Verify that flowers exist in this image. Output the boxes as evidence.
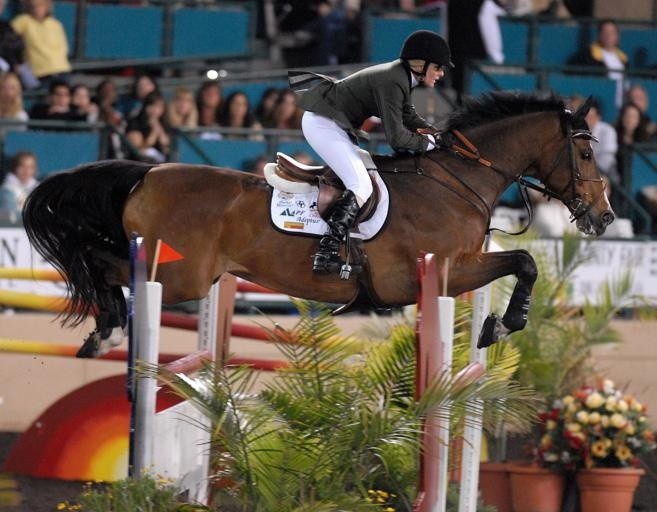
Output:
[529,377,656,474]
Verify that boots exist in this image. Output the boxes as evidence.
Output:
[312,189,362,275]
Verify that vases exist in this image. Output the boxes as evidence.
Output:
[575,464,645,512]
[505,462,566,511]
[479,458,512,512]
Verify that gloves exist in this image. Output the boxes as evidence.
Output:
[433,131,456,149]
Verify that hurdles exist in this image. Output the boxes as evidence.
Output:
[417,284,493,512]
[127,280,220,508]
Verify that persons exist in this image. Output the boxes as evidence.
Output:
[0,0,656,242]
[301,30,456,274]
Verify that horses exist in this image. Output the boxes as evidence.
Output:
[20,87,616,358]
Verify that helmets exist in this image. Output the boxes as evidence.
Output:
[401,30,455,68]
